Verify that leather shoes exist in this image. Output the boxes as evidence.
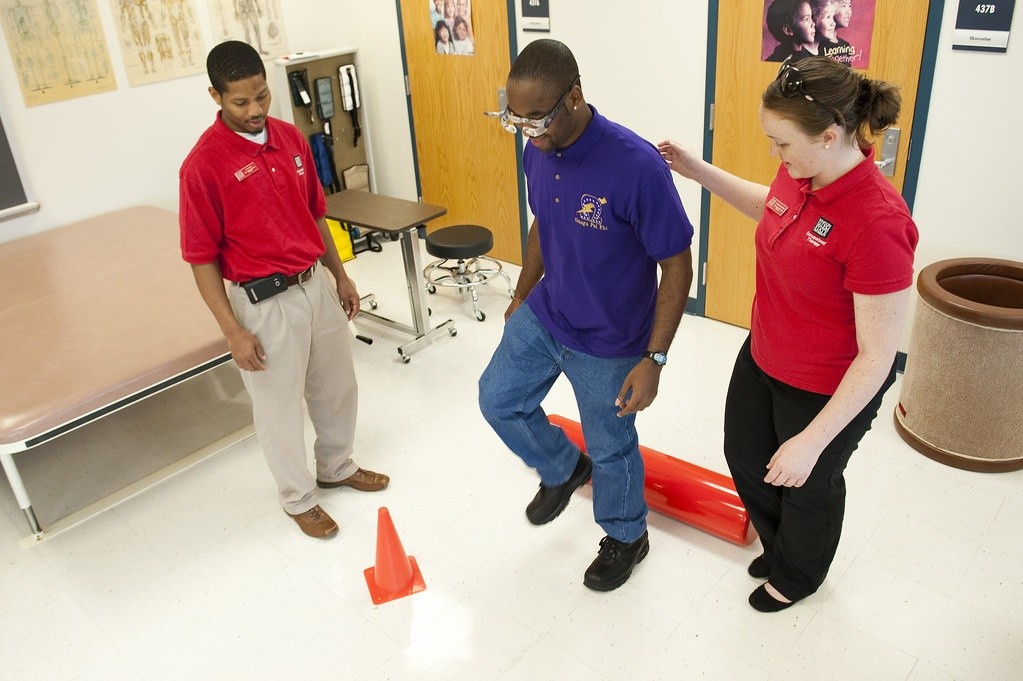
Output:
[284,505,339,539]
[526,453,592,524]
[316,467,390,491]
[583,529,649,591]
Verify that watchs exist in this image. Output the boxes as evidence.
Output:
[643,351,666,366]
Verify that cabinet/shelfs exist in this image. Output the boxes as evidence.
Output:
[273,46,385,255]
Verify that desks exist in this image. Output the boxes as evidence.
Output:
[1,206,261,547]
[324,189,457,363]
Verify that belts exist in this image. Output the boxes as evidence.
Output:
[234,261,318,289]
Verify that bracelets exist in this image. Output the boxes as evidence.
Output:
[509,289,525,302]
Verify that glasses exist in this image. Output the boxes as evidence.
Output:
[776,54,840,125]
[501,75,581,136]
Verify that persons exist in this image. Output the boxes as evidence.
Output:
[179,39,390,540]
[656,54,919,613]
[452,18,475,55]
[817,0,853,66]
[433,1,467,25]
[803,1,837,58]
[432,21,456,55]
[477,41,693,594]
[765,0,815,64]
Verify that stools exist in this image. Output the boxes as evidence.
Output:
[422,224,517,321]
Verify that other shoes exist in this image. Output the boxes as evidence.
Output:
[749,581,796,613]
[748,553,771,578]
